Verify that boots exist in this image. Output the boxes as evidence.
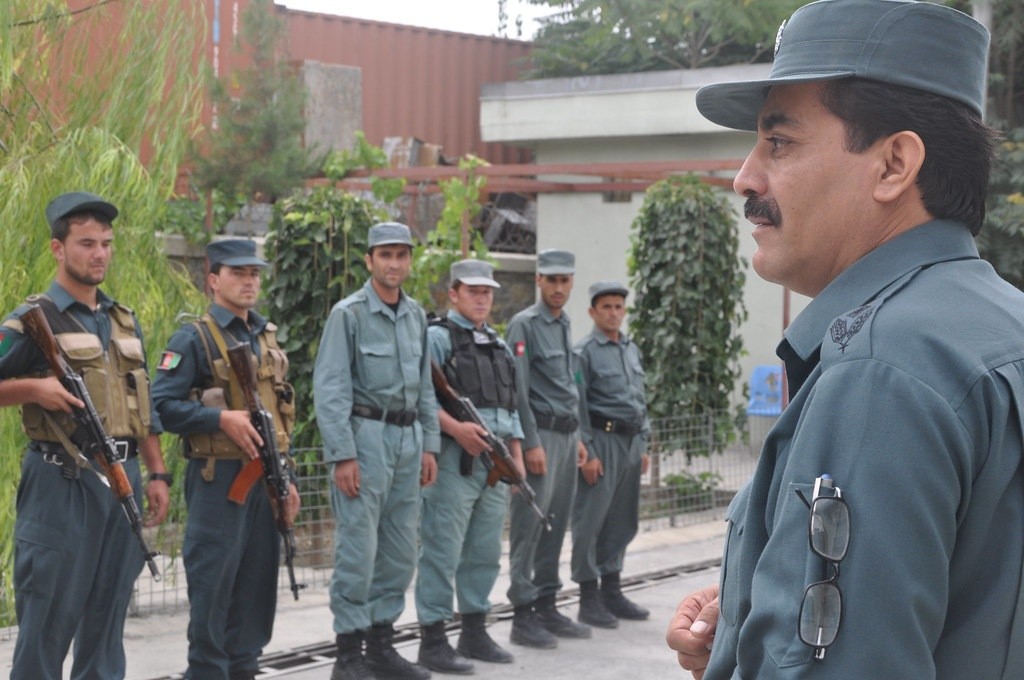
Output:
[510,604,559,647]
[363,623,431,680]
[578,581,620,629]
[329,628,380,680]
[600,574,649,620]
[456,613,515,664]
[418,620,473,674]
[535,594,592,639]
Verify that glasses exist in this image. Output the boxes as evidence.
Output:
[795,486,851,659]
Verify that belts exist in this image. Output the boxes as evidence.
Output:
[36,439,136,462]
[351,404,419,426]
[591,413,643,436]
[534,414,581,434]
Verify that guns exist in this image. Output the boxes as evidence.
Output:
[224,340,301,603]
[428,360,557,534]
[19,303,162,583]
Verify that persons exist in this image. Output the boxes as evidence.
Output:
[0,193,170,680]
[416,259,524,677]
[503,249,589,649]
[315,222,442,680]
[667,0,1024,679]
[574,281,651,628]
[151,239,301,680]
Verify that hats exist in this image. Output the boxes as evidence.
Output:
[695,0,991,130]
[367,223,416,251]
[451,260,500,288]
[45,192,119,230]
[589,282,628,306]
[206,239,269,266]
[537,250,576,275]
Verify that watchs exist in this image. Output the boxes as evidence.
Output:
[148,473,174,486]
